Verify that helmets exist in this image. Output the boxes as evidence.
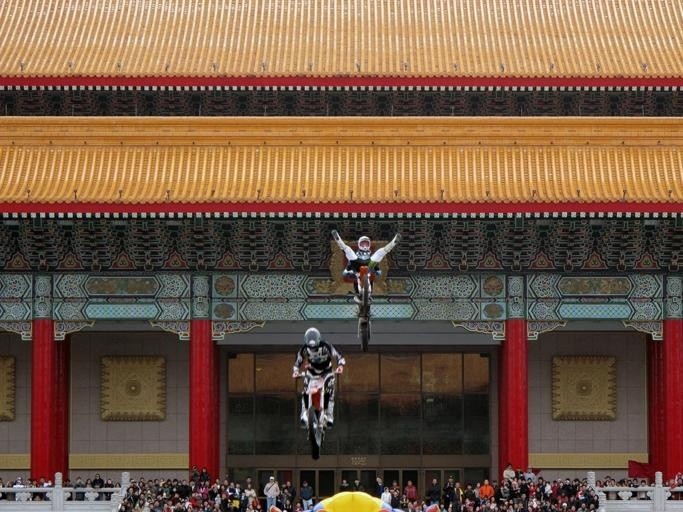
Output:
[358,236,370,251]
[304,328,320,347]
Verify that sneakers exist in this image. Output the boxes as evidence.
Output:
[332,230,341,241]
[394,234,401,244]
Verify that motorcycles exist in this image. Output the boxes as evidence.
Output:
[289,367,341,460]
[342,266,382,352]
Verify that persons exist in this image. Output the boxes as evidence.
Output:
[341,463,599,512]
[596,472,682,500]
[0,466,314,512]
[329,227,402,303]
[290,327,346,430]
